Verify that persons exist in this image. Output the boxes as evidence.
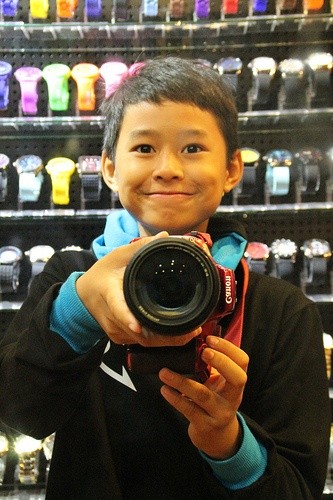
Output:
[0,54,333,500]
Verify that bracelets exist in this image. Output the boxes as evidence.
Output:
[303,0,325,11]
[0,0,21,19]
[278,0,296,12]
[111,0,130,20]
[85,0,104,19]
[55,0,78,17]
[252,0,270,14]
[192,0,210,20]
[140,0,159,17]
[29,0,50,19]
[221,0,240,16]
[168,0,186,18]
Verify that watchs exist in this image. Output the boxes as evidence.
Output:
[129,63,145,76]
[42,431,57,486]
[69,63,99,112]
[13,66,42,113]
[97,60,128,103]
[306,50,333,94]
[13,431,42,487]
[212,56,243,96]
[40,62,72,112]
[0,433,9,488]
[246,56,274,106]
[0,149,332,311]
[321,331,333,382]
[0,62,15,110]
[278,56,306,105]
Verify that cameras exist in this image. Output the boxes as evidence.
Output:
[116,234,238,384]
